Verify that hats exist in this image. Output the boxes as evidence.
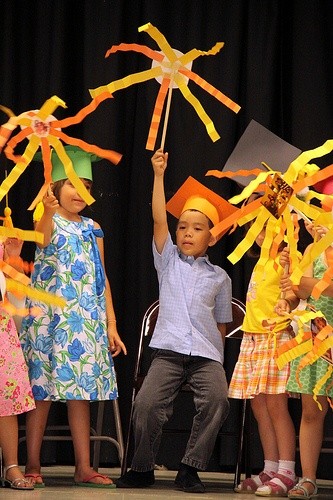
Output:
[31,145,103,183]
[166,176,241,246]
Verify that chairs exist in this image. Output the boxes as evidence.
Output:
[0,400,124,478]
[122,298,248,488]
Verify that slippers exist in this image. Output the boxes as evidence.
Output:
[75,473,117,488]
[24,473,45,488]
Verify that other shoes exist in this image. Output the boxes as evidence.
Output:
[173,467,206,492]
[115,470,155,488]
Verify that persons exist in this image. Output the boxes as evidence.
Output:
[226,191,301,497]
[115,148,239,493]
[0,227,37,489]
[24,145,128,488]
[274,174,333,498]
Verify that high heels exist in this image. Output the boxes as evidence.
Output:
[1,465,35,490]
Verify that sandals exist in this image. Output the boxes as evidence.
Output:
[233,471,276,493]
[254,473,299,497]
[288,478,319,499]
